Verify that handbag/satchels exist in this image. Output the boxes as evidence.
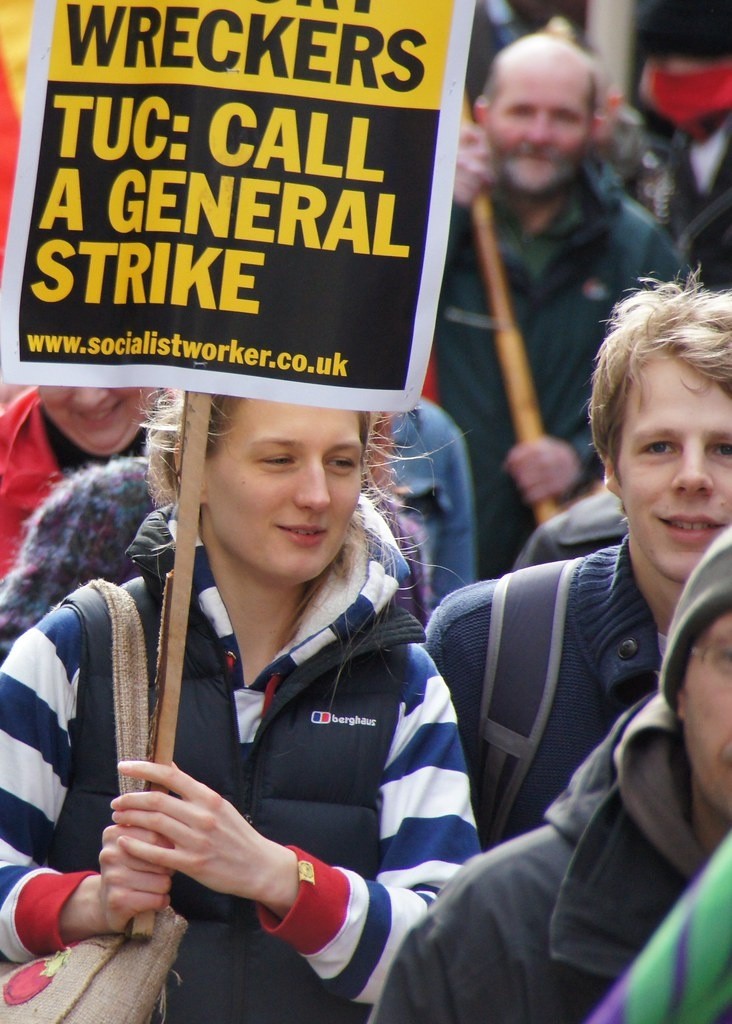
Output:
[0,576,187,1024]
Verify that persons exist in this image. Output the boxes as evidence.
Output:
[1,385,477,661]
[424,272,731,846]
[373,525,731,1024]
[432,31,702,573]
[0,390,473,1023]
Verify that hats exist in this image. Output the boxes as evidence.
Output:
[658,527,732,712]
[634,0,732,64]
